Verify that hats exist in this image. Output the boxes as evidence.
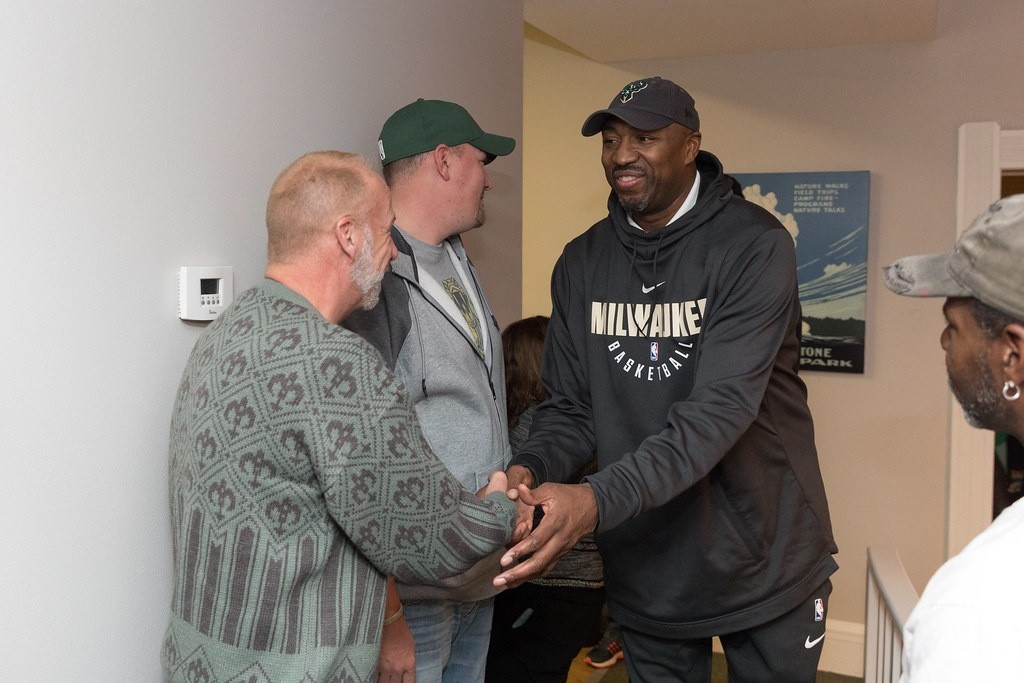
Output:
[377,98,516,166]
[882,194,1024,321]
[581,77,700,138]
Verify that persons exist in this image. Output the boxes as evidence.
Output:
[341,98,514,683]
[159,151,535,683]
[476,77,839,683]
[884,193,1024,683]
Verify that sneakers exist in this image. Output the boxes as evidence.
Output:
[584,622,624,667]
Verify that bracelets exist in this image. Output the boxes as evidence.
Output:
[383,602,403,627]
